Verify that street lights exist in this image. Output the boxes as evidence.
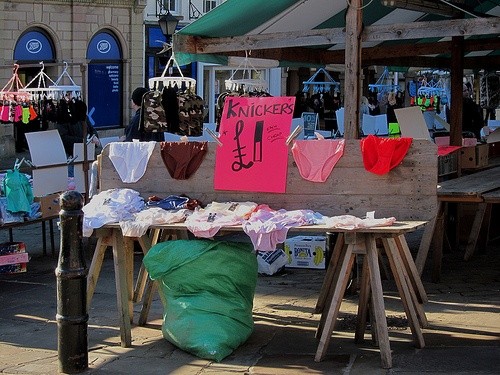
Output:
[159,10,180,76]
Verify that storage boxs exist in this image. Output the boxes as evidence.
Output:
[284,236,328,269]
[24,131,96,197]
[462,145,489,169]
[438,147,459,177]
[0,242,28,275]
[35,190,68,219]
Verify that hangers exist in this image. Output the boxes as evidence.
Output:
[417,78,446,97]
[224,50,270,93]
[148,52,197,89]
[303,68,341,93]
[368,66,402,94]
[0,61,82,105]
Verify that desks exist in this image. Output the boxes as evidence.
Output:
[415,167,500,278]
[57,222,428,369]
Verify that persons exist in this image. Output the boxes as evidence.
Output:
[123,87,166,142]
[462,84,483,132]
[57,115,101,159]
